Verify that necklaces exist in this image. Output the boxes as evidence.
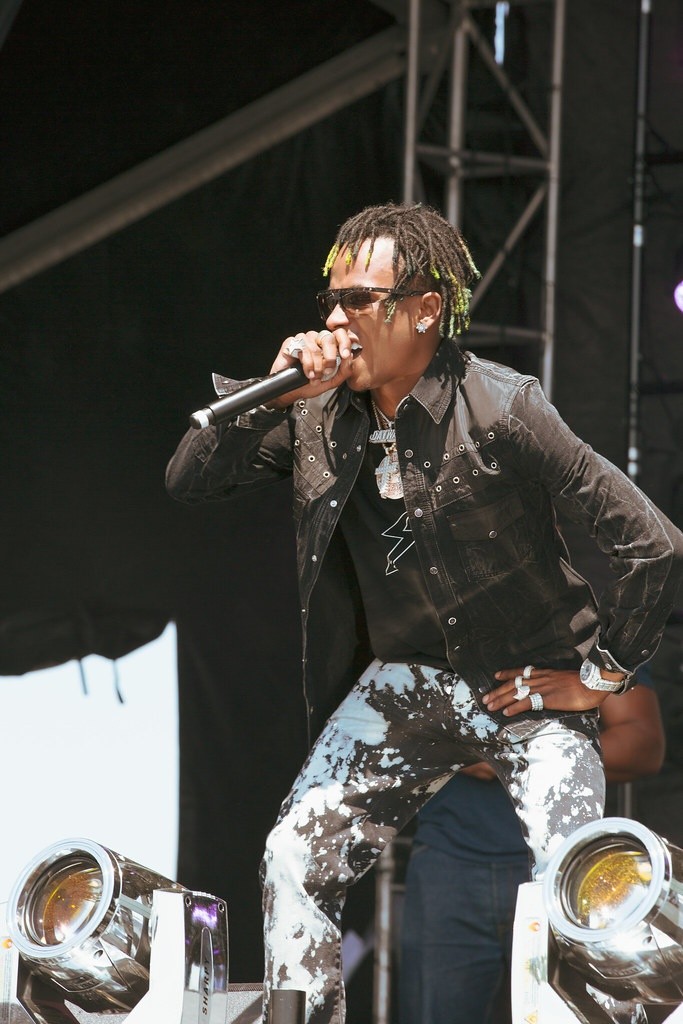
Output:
[368,399,405,499]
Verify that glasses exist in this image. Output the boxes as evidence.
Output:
[316,287,428,321]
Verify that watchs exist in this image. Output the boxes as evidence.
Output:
[580,658,624,692]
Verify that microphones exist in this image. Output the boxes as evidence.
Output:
[189,347,341,429]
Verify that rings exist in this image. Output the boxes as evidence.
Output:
[318,331,333,340]
[515,676,523,688]
[529,693,543,711]
[523,666,535,679]
[514,686,531,701]
[285,337,306,357]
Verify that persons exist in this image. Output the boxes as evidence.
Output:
[165,203,683,1024]
[396,509,665,1024]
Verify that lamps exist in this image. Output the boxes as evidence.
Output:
[510,817,683,1024]
[6,838,228,1024]
